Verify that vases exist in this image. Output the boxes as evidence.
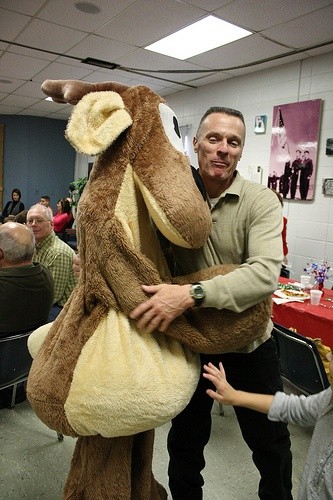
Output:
[316,281,324,290]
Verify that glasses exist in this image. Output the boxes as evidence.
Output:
[25,218,52,227]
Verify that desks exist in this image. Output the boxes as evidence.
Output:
[272,276,333,350]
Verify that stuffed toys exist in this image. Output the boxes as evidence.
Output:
[26,78,276,500]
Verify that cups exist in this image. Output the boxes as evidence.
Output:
[310,290,323,306]
[300,275,311,287]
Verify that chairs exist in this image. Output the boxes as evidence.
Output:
[272,322,330,396]
[0,329,64,442]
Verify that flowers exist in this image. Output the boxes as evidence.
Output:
[304,258,333,282]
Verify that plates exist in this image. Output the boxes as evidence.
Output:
[274,290,310,299]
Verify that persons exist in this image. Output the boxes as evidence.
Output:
[0,188,25,225]
[38,196,54,221]
[64,198,75,229]
[25,204,81,325]
[73,107,294,500]
[258,108,314,199]
[50,197,73,233]
[274,192,290,280]
[202,349,333,500]
[0,222,55,410]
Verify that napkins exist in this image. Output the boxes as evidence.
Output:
[287,281,314,289]
[273,297,304,305]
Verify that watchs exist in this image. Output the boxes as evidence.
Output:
[189,282,206,308]
